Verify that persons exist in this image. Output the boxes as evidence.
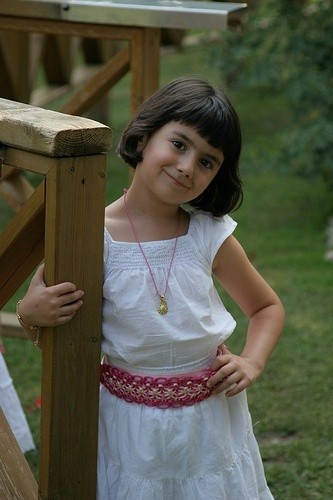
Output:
[16,79,285,500]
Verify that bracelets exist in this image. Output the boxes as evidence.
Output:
[16,300,40,347]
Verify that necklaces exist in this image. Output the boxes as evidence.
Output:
[123,189,180,315]
